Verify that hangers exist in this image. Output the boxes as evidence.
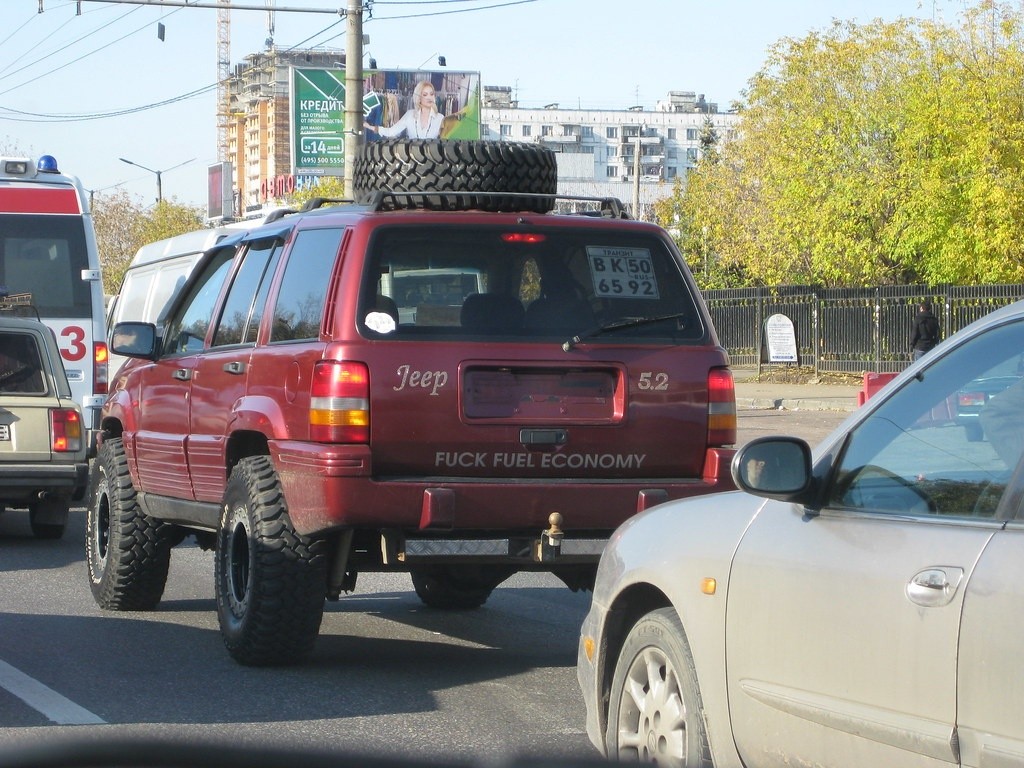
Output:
[370,88,457,102]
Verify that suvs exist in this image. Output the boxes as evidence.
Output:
[89,140,739,667]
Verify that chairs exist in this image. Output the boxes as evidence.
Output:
[461,293,523,329]
[367,293,398,333]
[525,281,597,335]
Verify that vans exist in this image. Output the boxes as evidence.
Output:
[1,154,249,500]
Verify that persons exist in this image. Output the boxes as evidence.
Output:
[908,300,941,361]
[745,457,766,488]
[363,81,445,140]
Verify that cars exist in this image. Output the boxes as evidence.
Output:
[0,319,89,540]
[578,298,1024,768]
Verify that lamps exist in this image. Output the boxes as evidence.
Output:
[418,51,446,71]
[362,52,377,69]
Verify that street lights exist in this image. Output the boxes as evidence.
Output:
[119,156,198,202]
